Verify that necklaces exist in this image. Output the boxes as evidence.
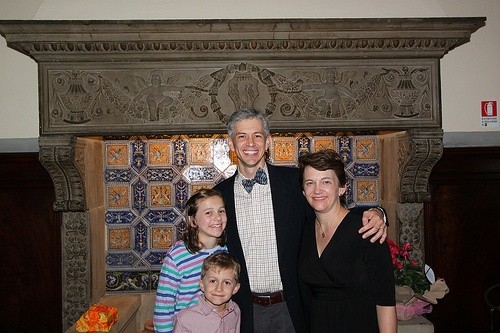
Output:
[317,209,341,239]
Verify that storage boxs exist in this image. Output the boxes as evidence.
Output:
[75,302,118,332]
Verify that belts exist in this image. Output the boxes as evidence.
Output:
[253,293,285,306]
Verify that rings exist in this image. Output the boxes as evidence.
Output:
[380,225,384,230]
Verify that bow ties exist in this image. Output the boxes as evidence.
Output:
[242,167,268,194]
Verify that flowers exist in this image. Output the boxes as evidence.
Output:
[387,238,431,295]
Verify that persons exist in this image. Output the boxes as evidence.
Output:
[140,106,390,333]
[172,251,242,333]
[152,188,229,333]
[298,147,400,333]
